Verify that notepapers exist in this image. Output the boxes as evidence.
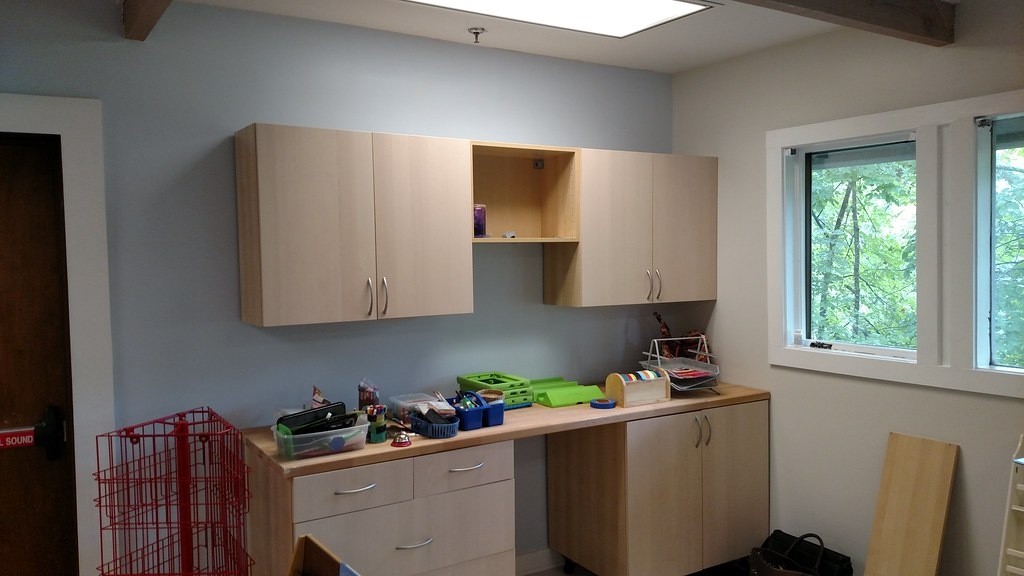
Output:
[427,401,456,416]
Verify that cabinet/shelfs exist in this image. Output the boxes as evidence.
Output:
[233,123,718,328]
[639,336,722,397]
[995,434,1024,576]
[91,405,255,576]
[239,380,772,576]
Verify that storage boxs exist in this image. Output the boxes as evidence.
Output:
[271,416,370,460]
[390,392,438,424]
[287,533,361,576]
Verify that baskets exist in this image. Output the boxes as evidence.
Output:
[410,413,460,438]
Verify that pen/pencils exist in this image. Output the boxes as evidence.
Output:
[433,389,456,411]
[357,383,380,410]
[455,390,480,407]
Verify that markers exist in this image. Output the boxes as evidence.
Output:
[363,404,387,422]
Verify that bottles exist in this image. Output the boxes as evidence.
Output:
[794,329,802,345]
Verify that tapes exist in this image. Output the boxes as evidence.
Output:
[455,403,464,409]
[461,399,474,408]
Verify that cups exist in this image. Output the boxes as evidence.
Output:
[365,419,386,443]
[474,203,487,237]
